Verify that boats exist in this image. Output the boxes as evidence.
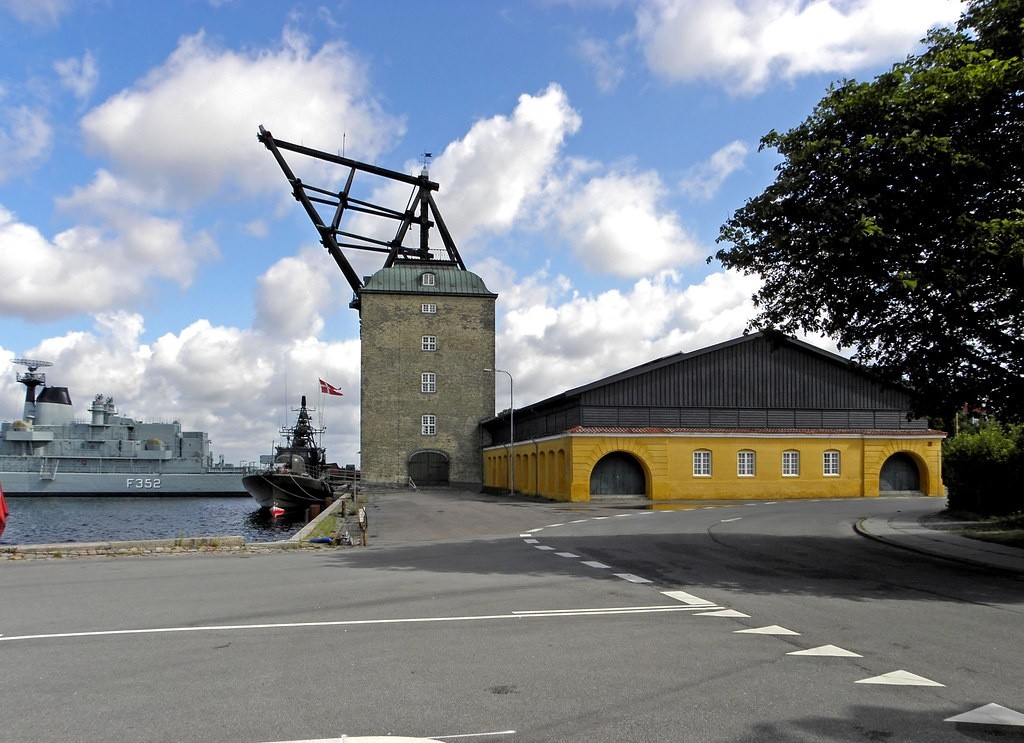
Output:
[241,395,336,522]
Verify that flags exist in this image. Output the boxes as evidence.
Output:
[319,379,344,396]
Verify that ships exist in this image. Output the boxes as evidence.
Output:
[1,357,277,496]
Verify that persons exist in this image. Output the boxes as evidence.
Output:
[264,463,276,471]
[284,464,290,474]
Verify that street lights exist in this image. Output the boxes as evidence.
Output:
[483,368,517,497]
